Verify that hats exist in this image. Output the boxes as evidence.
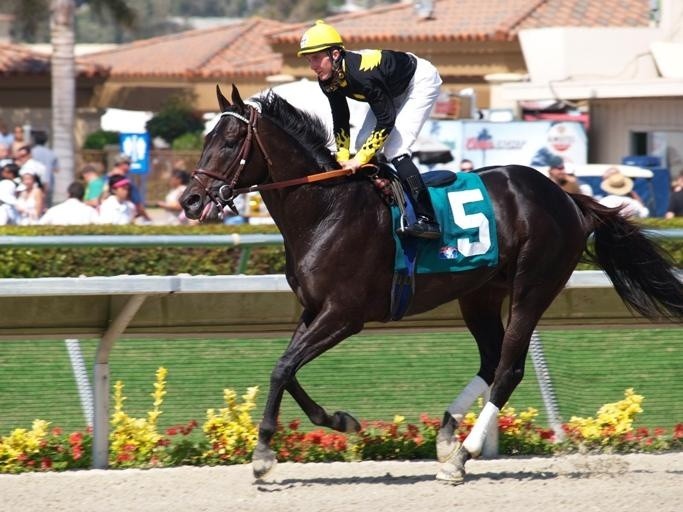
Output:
[110,174,129,188]
[599,173,635,196]
[296,23,345,59]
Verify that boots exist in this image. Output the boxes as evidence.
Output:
[396,172,441,240]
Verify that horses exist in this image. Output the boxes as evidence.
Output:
[176,79,683,486]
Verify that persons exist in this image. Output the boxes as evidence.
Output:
[295,19,444,241]
[456,155,683,221]
[0,120,278,229]
